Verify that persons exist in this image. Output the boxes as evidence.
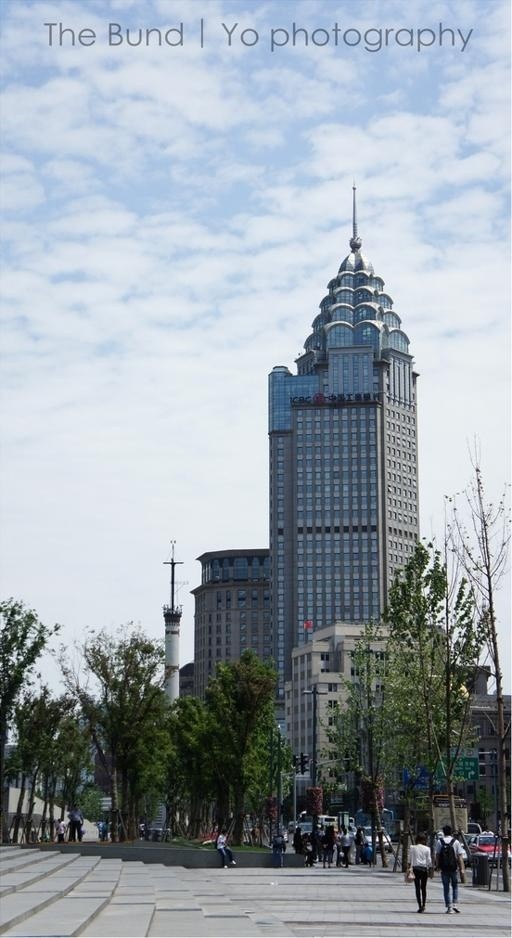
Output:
[432,826,465,914]
[251,825,260,847]
[407,834,433,913]
[56,818,67,842]
[68,803,84,842]
[66,820,76,841]
[216,829,237,869]
[269,834,286,867]
[97,819,146,841]
[293,822,372,868]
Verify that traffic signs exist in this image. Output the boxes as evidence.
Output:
[434,747,480,781]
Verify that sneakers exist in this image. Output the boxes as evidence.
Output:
[224,865,227,868]
[446,907,460,914]
[230,860,236,865]
[417,907,425,913]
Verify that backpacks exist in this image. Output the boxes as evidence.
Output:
[438,838,458,872]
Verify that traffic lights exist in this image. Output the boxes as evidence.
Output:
[293,755,301,774]
[301,753,310,776]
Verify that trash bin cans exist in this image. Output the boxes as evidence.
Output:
[472,852,488,888]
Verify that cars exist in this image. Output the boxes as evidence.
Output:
[428,822,511,870]
[242,808,394,853]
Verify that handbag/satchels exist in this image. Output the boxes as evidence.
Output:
[405,869,416,883]
